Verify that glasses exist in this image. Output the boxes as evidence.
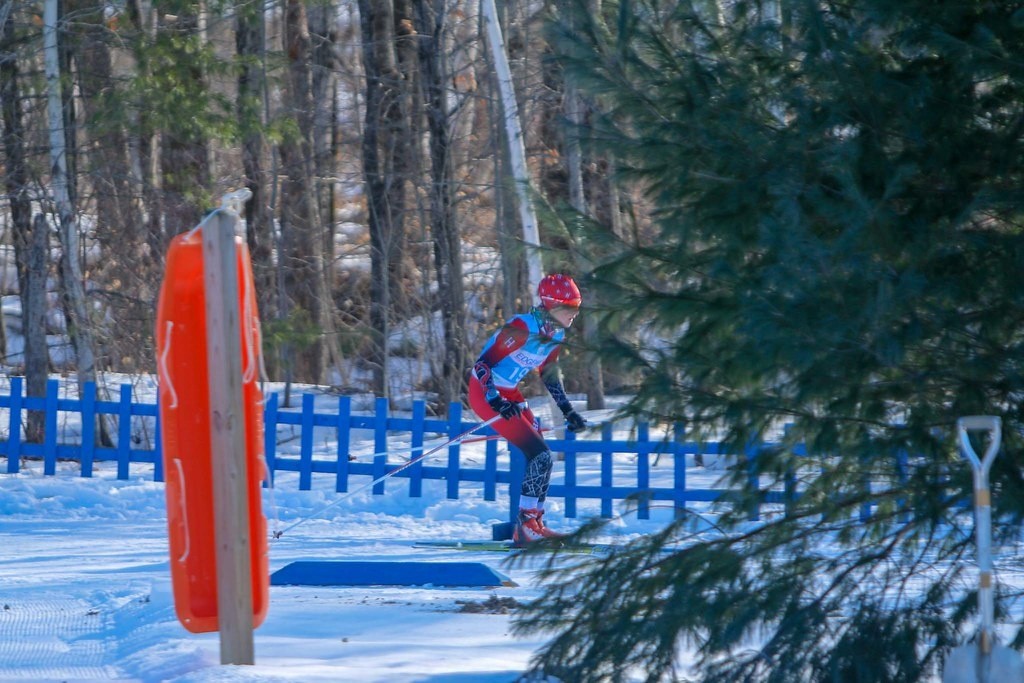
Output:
[563,299,581,311]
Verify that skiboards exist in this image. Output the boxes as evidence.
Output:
[413,541,653,558]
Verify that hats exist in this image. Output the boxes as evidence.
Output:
[539,275,581,311]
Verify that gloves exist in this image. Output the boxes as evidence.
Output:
[489,395,521,421]
[564,410,585,431]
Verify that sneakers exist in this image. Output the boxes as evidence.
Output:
[513,509,567,549]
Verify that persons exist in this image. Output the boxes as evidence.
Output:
[467,273,588,550]
